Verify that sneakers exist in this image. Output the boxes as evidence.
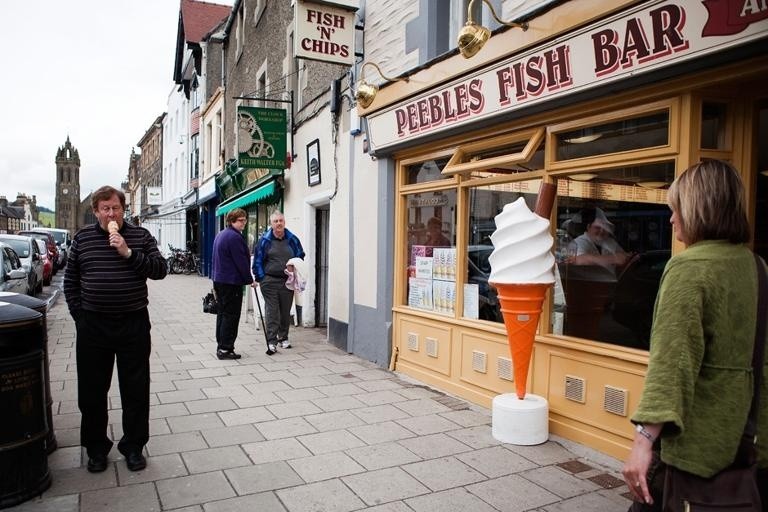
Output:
[278,339,291,348]
[266,343,276,353]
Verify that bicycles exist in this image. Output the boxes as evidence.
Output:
[164,244,210,278]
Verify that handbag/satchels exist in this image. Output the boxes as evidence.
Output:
[202,293,217,314]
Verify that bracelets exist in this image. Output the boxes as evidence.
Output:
[126,245,132,262]
[636,423,655,443]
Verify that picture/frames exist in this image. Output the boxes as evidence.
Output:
[306,138,322,187]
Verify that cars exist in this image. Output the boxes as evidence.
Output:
[447,209,680,353]
[0,224,75,302]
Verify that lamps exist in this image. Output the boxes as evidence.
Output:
[456,1,530,61]
[353,61,411,110]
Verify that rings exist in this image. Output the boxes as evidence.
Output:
[635,481,641,486]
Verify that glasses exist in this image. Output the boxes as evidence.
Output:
[237,219,248,223]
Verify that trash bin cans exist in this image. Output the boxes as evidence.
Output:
[0,291,57,510]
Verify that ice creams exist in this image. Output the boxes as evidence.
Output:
[487,198,556,398]
[109,220,119,234]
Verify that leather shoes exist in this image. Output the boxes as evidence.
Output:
[88,454,108,472]
[217,348,241,360]
[125,452,146,471]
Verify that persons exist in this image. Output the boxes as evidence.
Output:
[211,208,258,360]
[613,157,768,511]
[567,213,639,266]
[62,186,172,472]
[423,215,451,257]
[252,209,305,354]
